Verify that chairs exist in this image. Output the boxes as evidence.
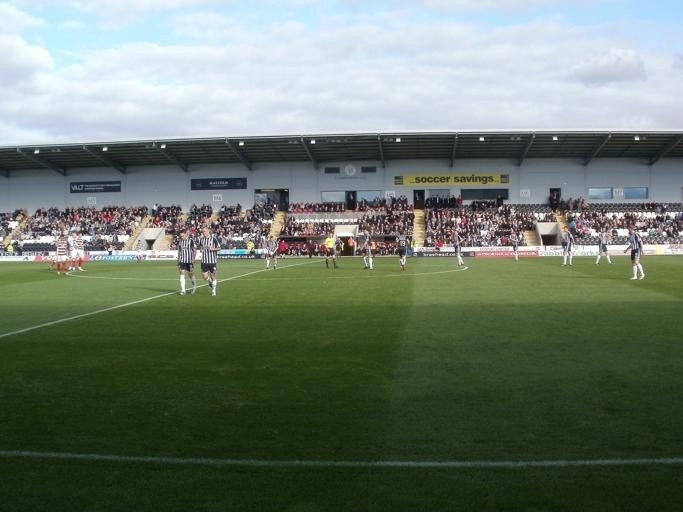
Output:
[535,212,547,220]
[296,218,357,224]
[572,211,683,238]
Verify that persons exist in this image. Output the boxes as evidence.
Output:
[198,225,221,298]
[545,212,557,222]
[280,239,324,257]
[148,203,185,250]
[450,229,465,266]
[210,198,278,258]
[594,225,614,265]
[623,225,645,280]
[314,221,334,235]
[71,232,88,271]
[324,231,339,269]
[266,235,279,269]
[289,201,345,212]
[68,229,78,271]
[425,194,536,250]
[396,235,409,271]
[186,203,213,236]
[363,243,374,269]
[281,216,308,236]
[0,206,148,256]
[568,211,683,245]
[559,225,575,266]
[133,239,144,264]
[642,201,667,212]
[355,194,415,255]
[169,227,198,295]
[550,194,589,212]
[54,234,69,274]
[336,236,354,255]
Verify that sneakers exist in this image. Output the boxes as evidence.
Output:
[629,274,645,280]
[211,289,216,297]
[179,292,186,296]
